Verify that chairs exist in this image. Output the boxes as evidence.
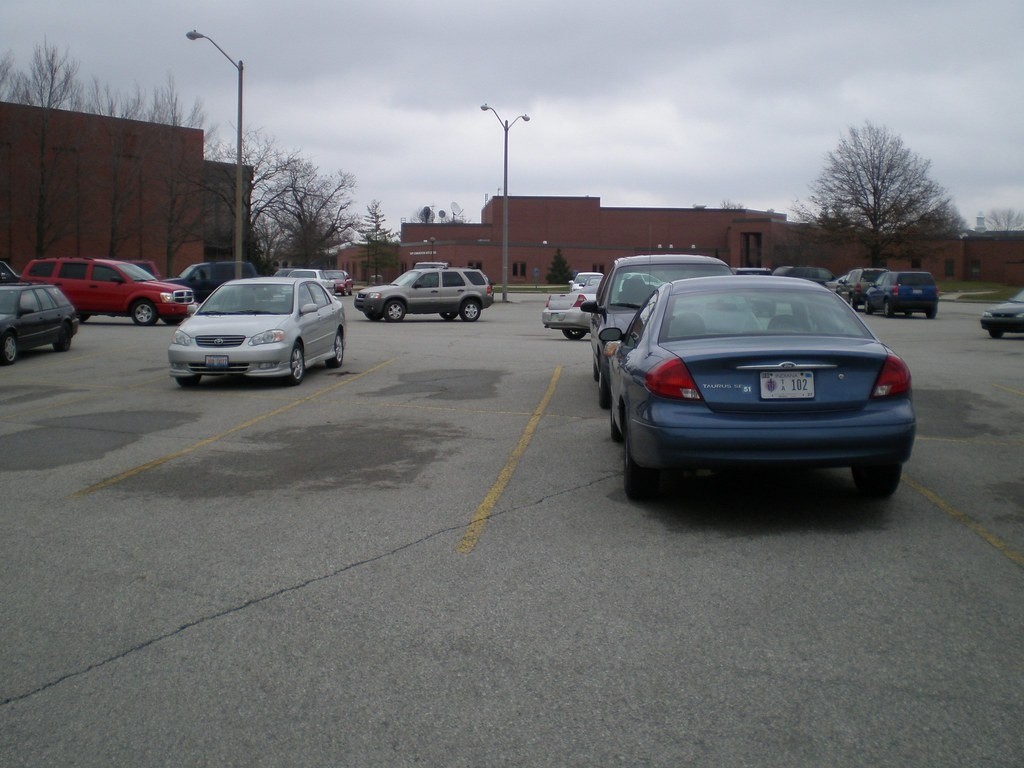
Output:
[667,311,707,338]
[617,278,649,306]
[767,314,806,333]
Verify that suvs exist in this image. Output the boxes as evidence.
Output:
[352,267,495,322]
[18,257,198,326]
[162,261,259,304]
[116,259,161,281]
[864,270,940,318]
[770,265,842,291]
[413,262,449,286]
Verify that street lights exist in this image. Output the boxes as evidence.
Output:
[186,30,244,284]
[430,236,436,265]
[481,104,530,302]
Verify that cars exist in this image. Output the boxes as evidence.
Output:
[542,281,626,339]
[619,272,668,294]
[835,267,890,311]
[580,253,772,407]
[0,285,80,365]
[0,259,20,285]
[168,276,347,386]
[597,275,918,504]
[826,275,846,294]
[567,272,603,293]
[272,268,354,297]
[579,275,605,289]
[980,288,1024,339]
[729,266,773,277]
